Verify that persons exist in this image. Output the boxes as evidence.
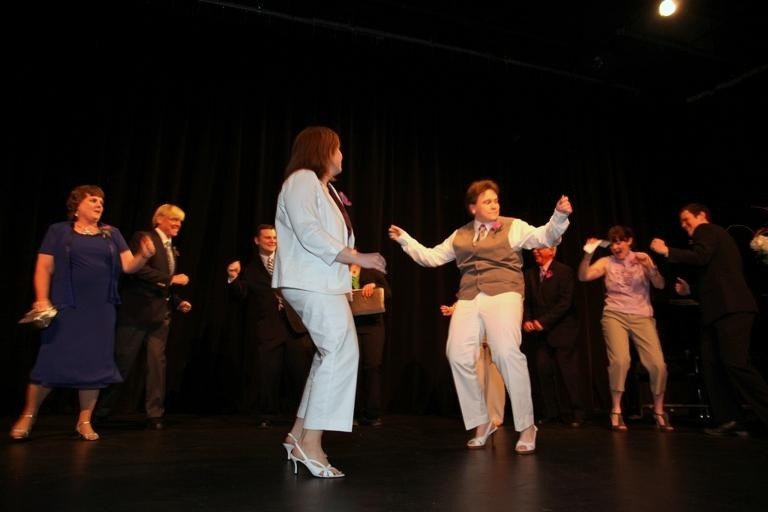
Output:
[10,183,155,442]
[650,202,758,436]
[269,124,387,479]
[439,300,509,427]
[71,203,192,436]
[226,224,307,430]
[387,179,573,455]
[579,223,677,434]
[348,247,393,427]
[522,244,583,426]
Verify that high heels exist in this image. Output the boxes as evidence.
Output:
[467,419,499,448]
[608,410,629,433]
[514,424,540,456]
[282,430,345,478]
[652,409,675,431]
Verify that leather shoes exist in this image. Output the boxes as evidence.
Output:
[702,419,752,440]
[146,417,164,430]
[552,415,581,430]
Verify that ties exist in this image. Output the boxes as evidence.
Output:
[266,257,285,312]
[476,224,487,242]
[166,241,174,276]
[539,266,545,283]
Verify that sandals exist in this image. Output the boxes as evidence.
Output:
[10,413,37,440]
[74,420,101,441]
